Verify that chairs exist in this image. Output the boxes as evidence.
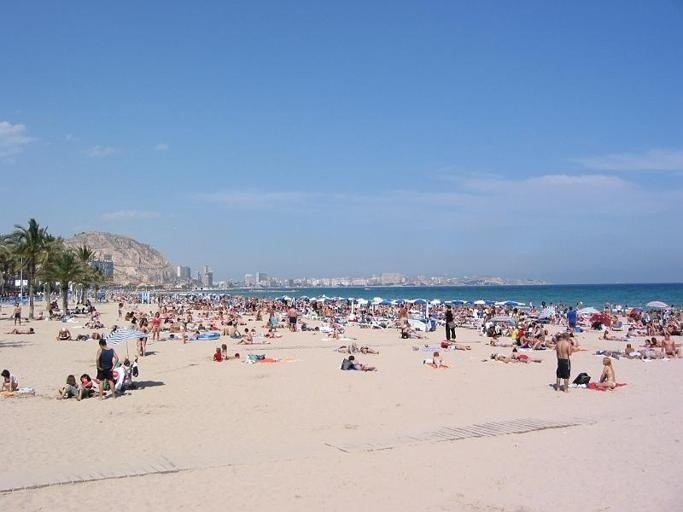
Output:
[305,314,391,329]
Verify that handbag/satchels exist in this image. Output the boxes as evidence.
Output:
[448,321,456,329]
[573,373,591,384]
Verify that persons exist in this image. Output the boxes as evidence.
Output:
[1,274,683,402]
